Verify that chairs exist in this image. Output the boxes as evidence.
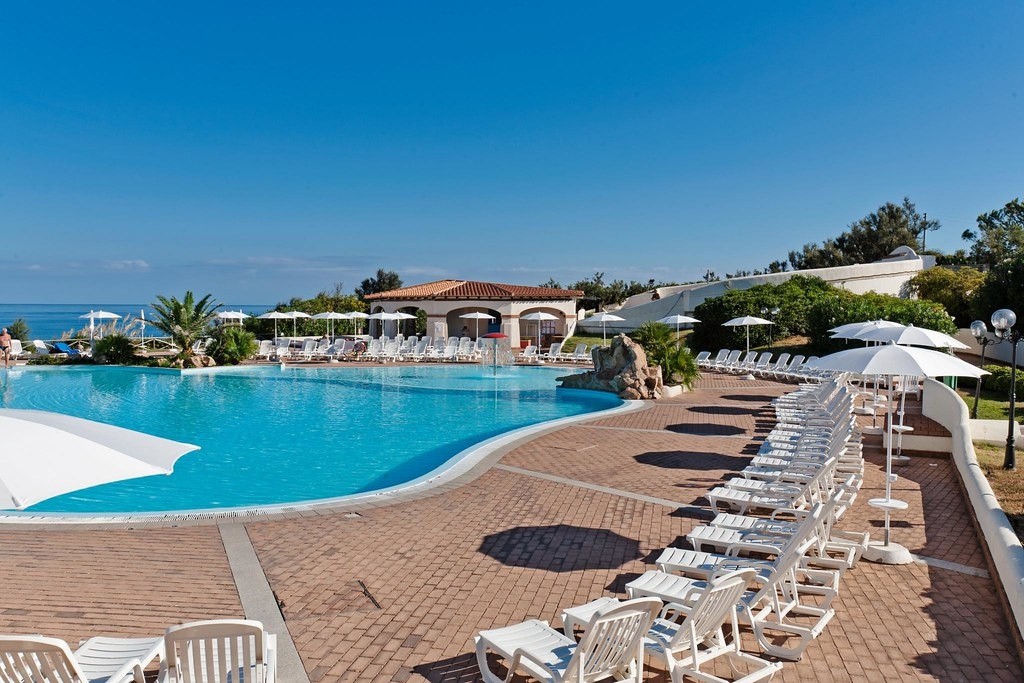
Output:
[0,336,920,683]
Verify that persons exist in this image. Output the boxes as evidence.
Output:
[0,328,13,368]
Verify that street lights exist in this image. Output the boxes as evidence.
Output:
[991,308,1024,470]
[969,320,1012,419]
[761,307,781,349]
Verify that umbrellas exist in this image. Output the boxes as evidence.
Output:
[218,309,252,332]
[255,310,417,359]
[656,314,701,352]
[829,318,971,503]
[800,340,991,546]
[0,409,201,510]
[587,314,625,347]
[80,310,121,341]
[459,310,496,349]
[721,315,776,373]
[520,311,559,355]
